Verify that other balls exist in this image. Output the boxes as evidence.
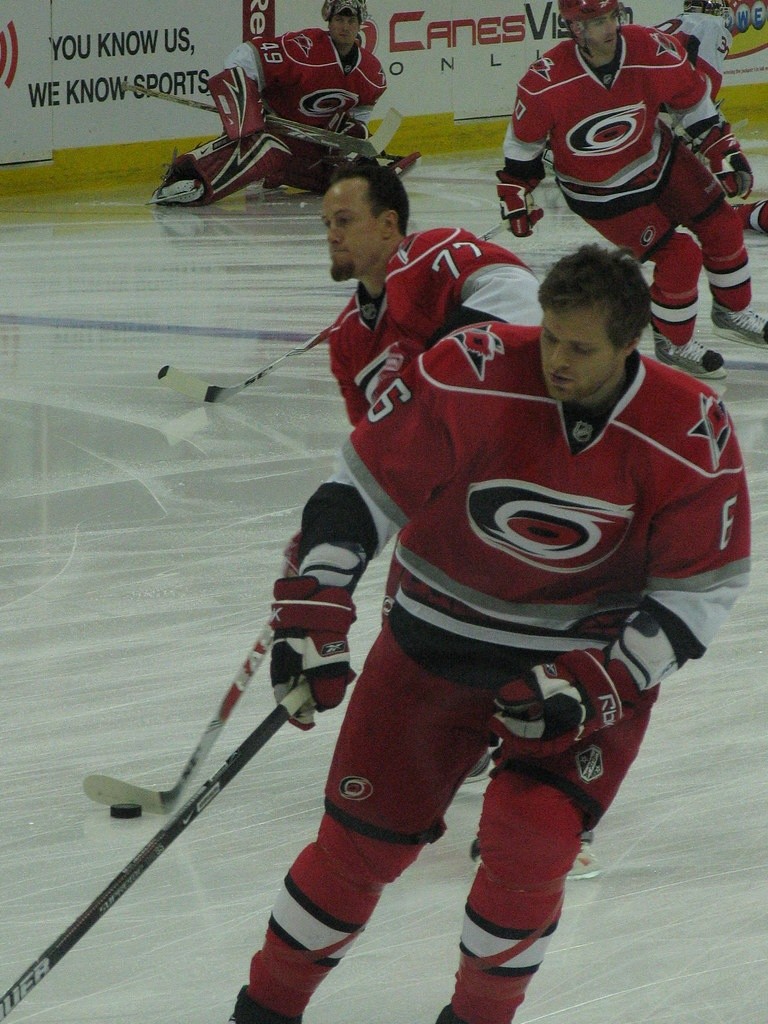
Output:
[109,803,143,819]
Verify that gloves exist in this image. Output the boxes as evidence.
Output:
[497,170,544,236]
[698,121,754,199]
[269,576,357,731]
[487,648,640,763]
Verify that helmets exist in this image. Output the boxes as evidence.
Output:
[559,0,625,20]
[684,0,728,16]
[323,0,368,22]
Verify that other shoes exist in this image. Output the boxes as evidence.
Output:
[654,332,728,381]
[710,300,768,346]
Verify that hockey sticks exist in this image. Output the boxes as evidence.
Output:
[156,219,510,404]
[81,604,280,816]
[0,678,312,1024]
[120,78,405,160]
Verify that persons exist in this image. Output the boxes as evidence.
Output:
[496,0,768,380]
[147,0,422,208]
[228,242,752,1024]
[319,160,598,882]
